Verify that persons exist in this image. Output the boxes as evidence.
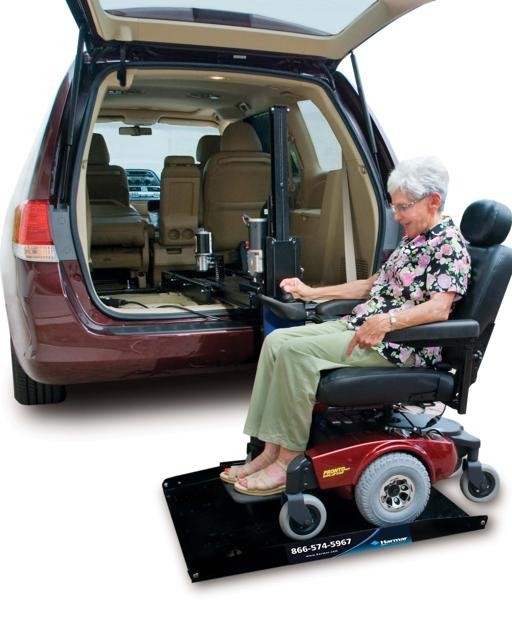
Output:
[215,154,471,494]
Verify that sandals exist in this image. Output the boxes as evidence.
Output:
[234,460,292,496]
[219,456,271,484]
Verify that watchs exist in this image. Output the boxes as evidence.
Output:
[388,313,396,331]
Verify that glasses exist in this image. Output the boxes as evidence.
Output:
[387,197,425,214]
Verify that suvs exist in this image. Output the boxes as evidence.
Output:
[0,0,440,404]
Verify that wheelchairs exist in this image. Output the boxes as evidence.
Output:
[217,195,511,546]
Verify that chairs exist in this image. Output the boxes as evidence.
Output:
[195,135,223,167]
[202,125,272,251]
[87,133,131,204]
